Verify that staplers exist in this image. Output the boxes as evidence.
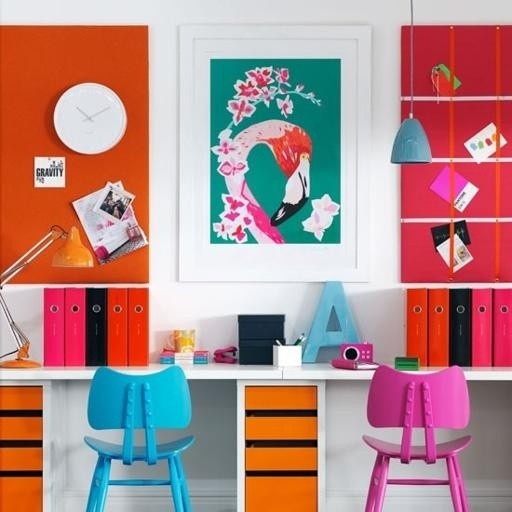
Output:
[213,346,238,363]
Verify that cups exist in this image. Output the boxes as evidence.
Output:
[166,327,196,354]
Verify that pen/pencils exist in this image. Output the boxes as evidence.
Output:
[295,332,306,345]
[275,339,283,346]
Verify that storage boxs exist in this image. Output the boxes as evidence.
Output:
[238,314,286,365]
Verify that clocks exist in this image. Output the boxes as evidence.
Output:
[53,82,127,155]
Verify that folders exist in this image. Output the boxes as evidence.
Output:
[404,286,511,367]
[41,286,150,367]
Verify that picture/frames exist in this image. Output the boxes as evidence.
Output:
[177,21,375,285]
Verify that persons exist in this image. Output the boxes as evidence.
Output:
[100,191,132,219]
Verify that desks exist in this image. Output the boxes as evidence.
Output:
[0,362,512,512]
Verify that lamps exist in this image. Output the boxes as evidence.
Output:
[0,224,95,370]
[390,1,433,166]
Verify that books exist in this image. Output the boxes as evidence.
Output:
[159,350,209,365]
[331,359,380,370]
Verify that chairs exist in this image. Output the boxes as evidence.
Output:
[361,365,473,511]
[80,364,198,512]
